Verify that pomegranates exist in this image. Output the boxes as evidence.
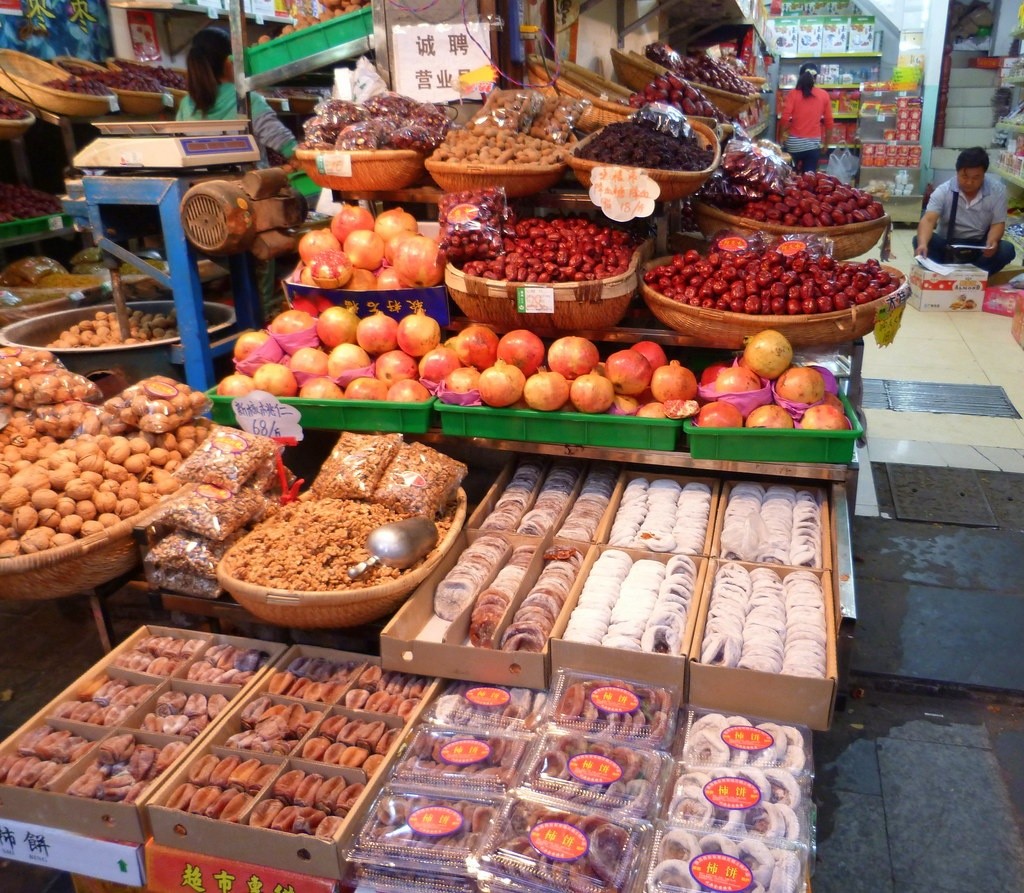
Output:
[215,306,855,431]
[293,203,444,290]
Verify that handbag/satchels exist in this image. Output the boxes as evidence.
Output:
[827,139,860,184]
[944,239,992,273]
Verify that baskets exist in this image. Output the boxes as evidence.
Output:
[296,44,912,347]
[218,488,468,628]
[0,480,199,602]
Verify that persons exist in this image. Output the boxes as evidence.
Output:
[780,63,834,173]
[177,27,296,168]
[913,147,1016,276]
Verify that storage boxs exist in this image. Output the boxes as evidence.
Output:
[760,0,1024,177]
[0,473,838,893]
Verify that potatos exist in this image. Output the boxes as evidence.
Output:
[257,0,371,47]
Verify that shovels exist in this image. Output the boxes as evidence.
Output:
[349,516,437,578]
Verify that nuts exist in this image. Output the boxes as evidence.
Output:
[230,430,468,592]
[0,345,219,558]
[145,427,287,600]
[430,90,590,167]
[46,307,181,350]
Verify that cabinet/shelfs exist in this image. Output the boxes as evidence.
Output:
[988,30,1024,258]
[857,82,922,223]
[780,52,881,183]
[616,0,770,140]
[164,1,316,118]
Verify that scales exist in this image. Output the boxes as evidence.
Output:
[72,121,261,177]
